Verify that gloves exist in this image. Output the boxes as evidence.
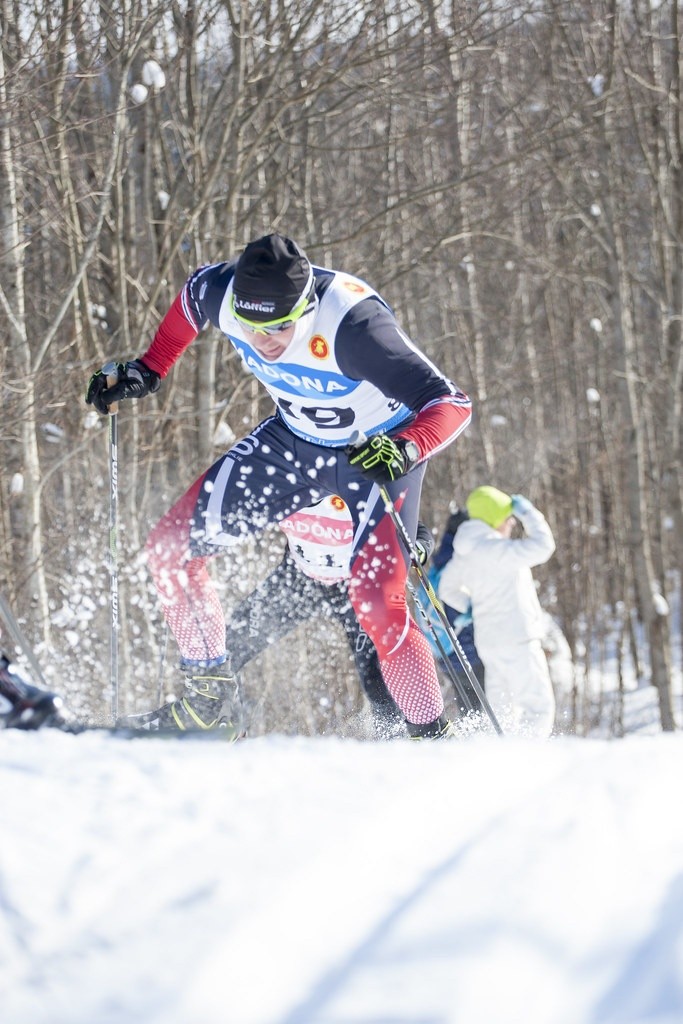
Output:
[510,494,528,516]
[349,434,418,484]
[85,359,160,414]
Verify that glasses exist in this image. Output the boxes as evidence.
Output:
[230,294,308,336]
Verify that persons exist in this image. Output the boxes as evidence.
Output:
[217,494,407,741]
[416,485,571,741]
[85,232,472,741]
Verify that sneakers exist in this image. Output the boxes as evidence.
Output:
[116,657,243,743]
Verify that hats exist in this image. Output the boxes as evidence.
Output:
[232,234,314,322]
[466,485,513,529]
